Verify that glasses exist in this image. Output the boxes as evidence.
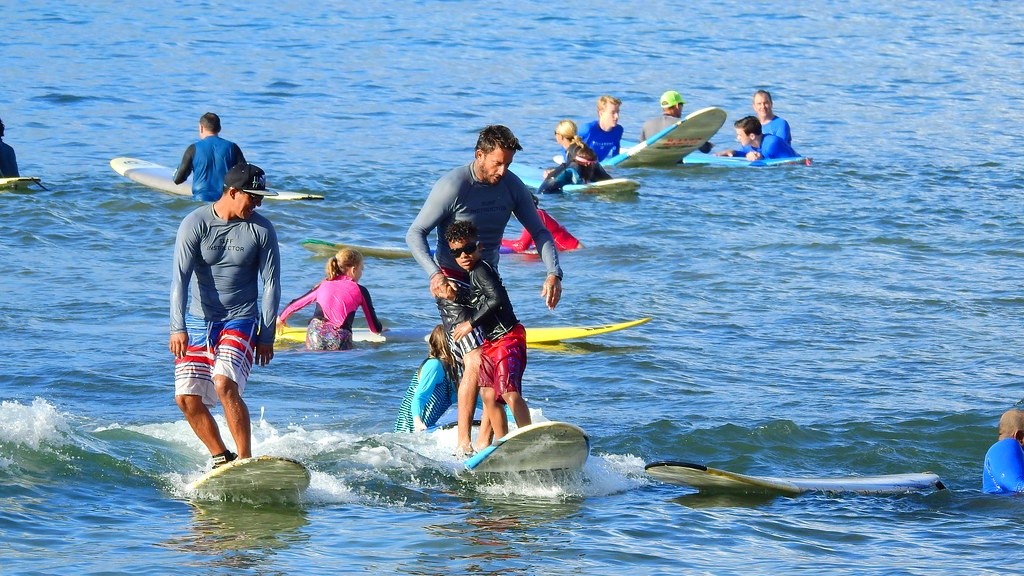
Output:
[450,240,479,259]
[554,131,559,135]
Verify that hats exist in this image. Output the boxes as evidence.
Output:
[660,91,686,108]
[223,163,279,195]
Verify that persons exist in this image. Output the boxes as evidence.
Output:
[715,90,802,162]
[502,190,585,253]
[275,248,382,352]
[393,126,563,459]
[169,164,281,469]
[536,96,623,195]
[0,118,19,181]
[173,112,247,202]
[640,90,714,164]
[982,409,1024,496]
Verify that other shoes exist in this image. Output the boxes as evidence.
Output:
[212,450,238,469]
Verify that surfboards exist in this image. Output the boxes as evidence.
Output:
[300,238,535,260]
[389,421,591,472]
[619,139,814,167]
[645,460,941,494]
[189,455,311,495]
[552,106,727,167]
[109,156,324,201]
[275,317,653,343]
[507,162,641,195]
[0,177,41,190]
[426,403,483,433]
